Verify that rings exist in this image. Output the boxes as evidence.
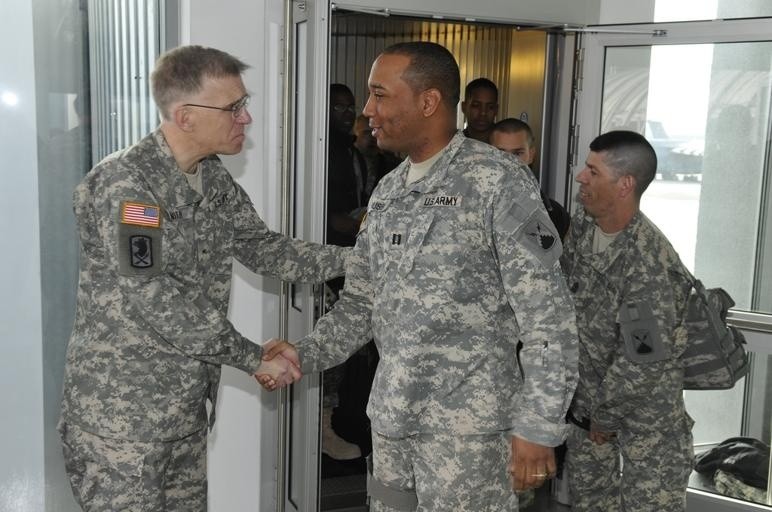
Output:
[537,472,547,480]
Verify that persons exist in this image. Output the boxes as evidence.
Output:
[256,41,579,512]
[328,82,368,376]
[322,282,362,460]
[554,130,699,511]
[460,77,500,145]
[488,118,572,248]
[55,46,355,510]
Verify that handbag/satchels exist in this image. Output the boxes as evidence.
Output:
[683,287,754,390]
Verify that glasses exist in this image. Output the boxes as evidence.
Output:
[181,93,252,121]
[331,102,356,115]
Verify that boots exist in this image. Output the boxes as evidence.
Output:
[322,406,362,461]
